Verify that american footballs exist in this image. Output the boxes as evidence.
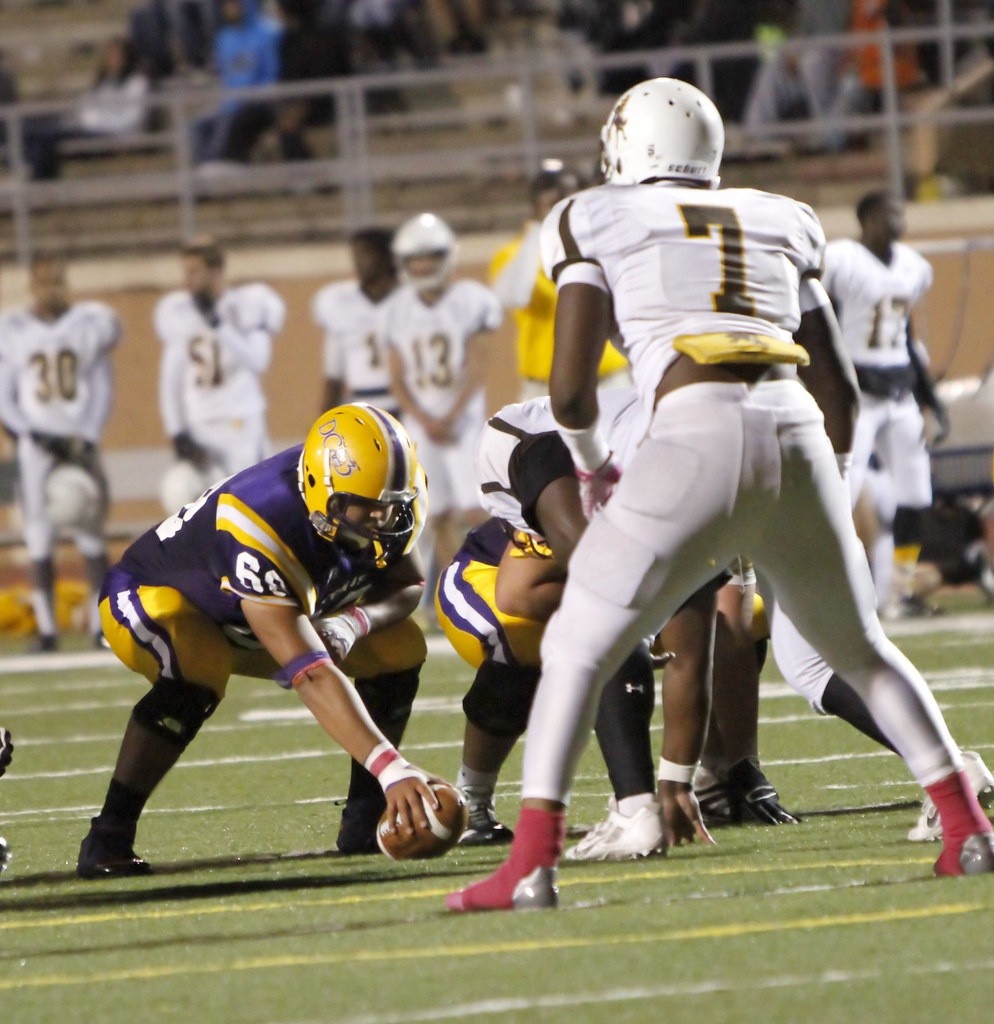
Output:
[376,781,468,862]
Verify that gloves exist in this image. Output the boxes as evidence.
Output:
[723,757,802,828]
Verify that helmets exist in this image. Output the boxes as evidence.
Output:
[599,77,725,190]
[298,402,420,568]
[392,212,459,289]
[530,152,585,220]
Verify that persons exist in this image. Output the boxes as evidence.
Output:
[1,1,994,653]
[446,78,994,912]
[77,401,454,875]
[432,388,994,863]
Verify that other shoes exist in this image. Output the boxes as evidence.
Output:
[444,863,561,913]
[933,831,994,877]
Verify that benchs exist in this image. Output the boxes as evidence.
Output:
[0,14,994,240]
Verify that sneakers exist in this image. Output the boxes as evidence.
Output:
[907,750,994,842]
[455,802,514,846]
[692,780,762,829]
[564,791,668,861]
[333,792,383,853]
[76,816,154,879]
